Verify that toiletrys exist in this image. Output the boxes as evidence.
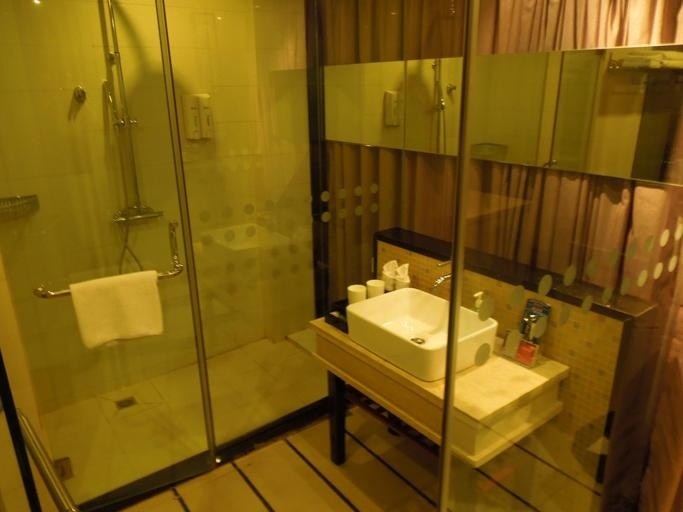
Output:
[182,94,202,141]
[197,93,214,139]
[472,292,484,311]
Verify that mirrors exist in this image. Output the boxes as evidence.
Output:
[324,56,462,155]
[469,47,682,189]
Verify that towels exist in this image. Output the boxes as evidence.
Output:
[620,49,682,71]
[69,271,165,349]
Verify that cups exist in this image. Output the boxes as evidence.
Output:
[347,284,367,304]
[366,280,386,298]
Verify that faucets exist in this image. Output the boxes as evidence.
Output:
[431,259,453,287]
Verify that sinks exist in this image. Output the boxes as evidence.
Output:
[344,287,499,383]
[206,223,291,259]
